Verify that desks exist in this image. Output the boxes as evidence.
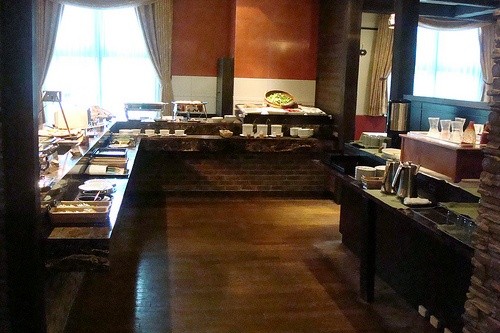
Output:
[331,172,476,333]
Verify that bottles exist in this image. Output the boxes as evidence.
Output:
[462,120,476,144]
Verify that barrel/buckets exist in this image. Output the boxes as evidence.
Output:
[386,98,411,135]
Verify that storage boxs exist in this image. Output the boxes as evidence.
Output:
[399,134,482,183]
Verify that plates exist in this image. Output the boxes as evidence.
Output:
[144,134,187,137]
[240,134,283,136]
[355,148,402,184]
[241,107,331,117]
[404,197,432,206]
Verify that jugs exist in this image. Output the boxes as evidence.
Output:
[381,158,421,199]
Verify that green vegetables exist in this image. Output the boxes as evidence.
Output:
[266,92,292,104]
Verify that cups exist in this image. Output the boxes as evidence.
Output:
[298,128,314,138]
[290,127,302,138]
[242,123,253,135]
[119,129,184,135]
[271,124,282,135]
[428,117,483,145]
[257,124,268,135]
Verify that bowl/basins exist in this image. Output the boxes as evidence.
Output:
[264,90,295,108]
[360,176,384,189]
[219,129,233,138]
[225,115,236,124]
[212,117,223,123]
[77,178,118,201]
